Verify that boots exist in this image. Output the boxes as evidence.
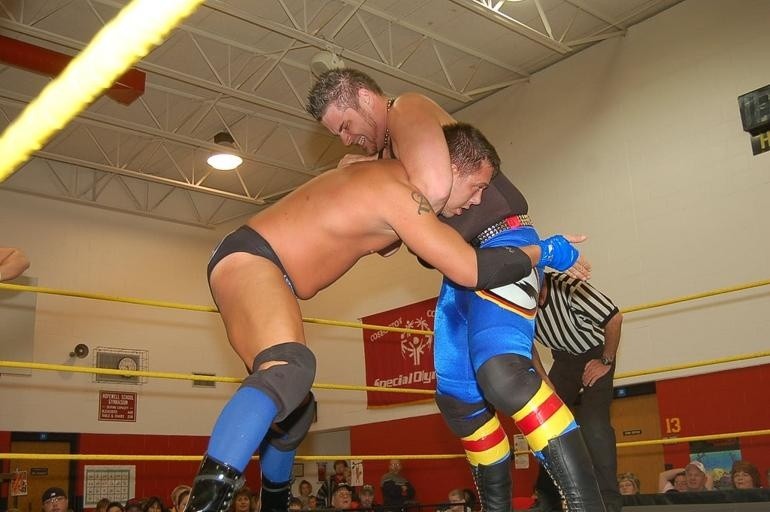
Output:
[465,455,516,512]
[183,453,242,512]
[530,429,607,511]
[262,471,292,511]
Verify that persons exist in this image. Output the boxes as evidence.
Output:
[231,487,256,511]
[43,486,192,511]
[446,489,477,511]
[616,473,641,495]
[181,123,592,510]
[658,460,717,494]
[533,270,623,511]
[289,459,416,510]
[731,462,760,489]
[305,67,607,512]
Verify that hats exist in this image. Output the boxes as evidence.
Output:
[43,486,66,502]
[330,482,354,491]
[685,459,706,476]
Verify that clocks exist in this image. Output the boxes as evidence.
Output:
[116,358,141,380]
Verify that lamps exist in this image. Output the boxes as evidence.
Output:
[307,46,349,86]
[205,131,245,172]
[67,343,90,359]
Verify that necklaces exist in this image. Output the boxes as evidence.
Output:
[383,98,394,146]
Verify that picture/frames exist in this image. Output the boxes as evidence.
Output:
[292,463,305,478]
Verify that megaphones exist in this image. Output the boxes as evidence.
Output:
[74,344,89,358]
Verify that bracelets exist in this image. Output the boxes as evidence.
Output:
[601,357,612,367]
